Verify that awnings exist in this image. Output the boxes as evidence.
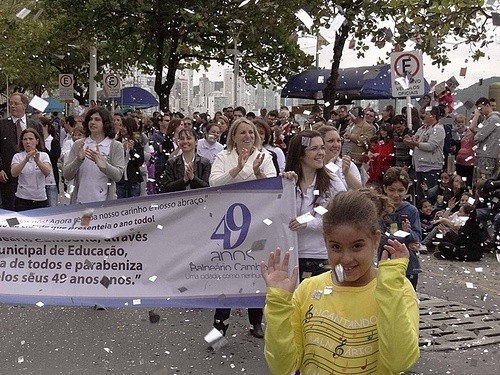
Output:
[84,86,159,112]
[280,64,432,115]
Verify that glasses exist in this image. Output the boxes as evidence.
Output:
[365,113,375,117]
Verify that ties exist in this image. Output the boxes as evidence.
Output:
[16,119,24,153]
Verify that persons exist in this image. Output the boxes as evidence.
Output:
[0,92,45,211]
[163,128,211,192]
[278,129,348,284]
[260,186,422,375]
[380,166,421,290]
[317,125,362,189]
[62,107,125,205]
[208,117,277,339]
[10,128,52,212]
[29,96,500,263]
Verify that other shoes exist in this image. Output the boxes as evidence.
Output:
[417,243,427,254]
[433,251,449,260]
[249,324,264,338]
